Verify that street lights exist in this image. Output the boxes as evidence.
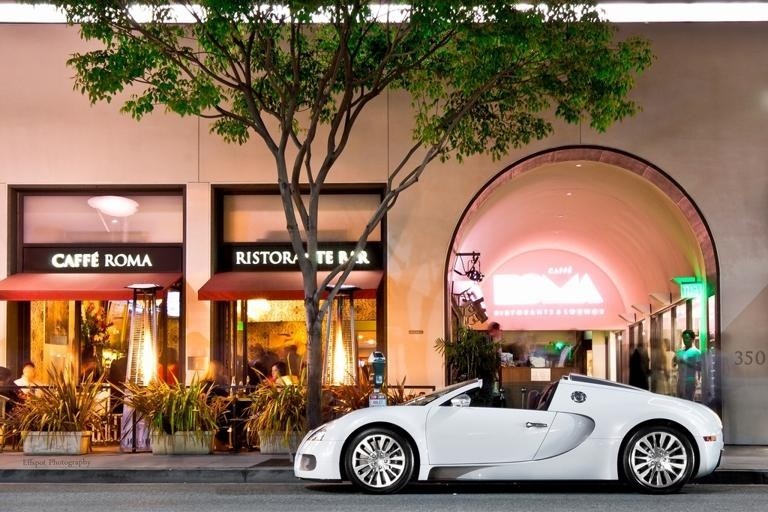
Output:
[320,282,363,386]
[119,282,165,451]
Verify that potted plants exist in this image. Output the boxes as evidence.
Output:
[241,352,307,454]
[105,371,237,454]
[0,362,114,455]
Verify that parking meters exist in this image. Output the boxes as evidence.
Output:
[365,347,388,406]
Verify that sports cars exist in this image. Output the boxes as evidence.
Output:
[287,365,734,496]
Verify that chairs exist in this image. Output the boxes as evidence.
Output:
[527,382,554,409]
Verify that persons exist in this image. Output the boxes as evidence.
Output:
[264,360,286,385]
[159,347,180,386]
[109,340,128,415]
[228,342,273,454]
[0,365,23,410]
[479,320,502,407]
[672,329,703,401]
[15,362,43,395]
[627,341,654,391]
